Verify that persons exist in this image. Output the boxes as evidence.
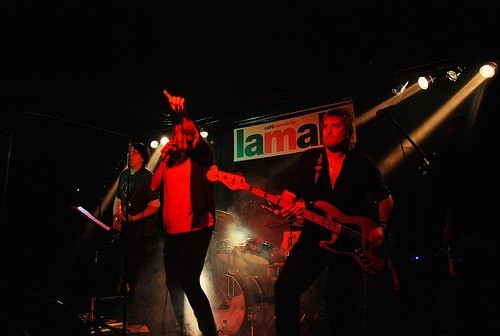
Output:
[91,142,161,315]
[149,89,218,336]
[273,109,394,336]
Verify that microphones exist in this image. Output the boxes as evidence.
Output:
[162,143,178,161]
[377,105,399,120]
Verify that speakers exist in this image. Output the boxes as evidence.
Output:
[0,292,90,336]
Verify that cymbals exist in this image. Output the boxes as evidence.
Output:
[216,210,235,226]
[213,218,228,234]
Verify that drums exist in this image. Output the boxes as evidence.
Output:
[241,238,278,265]
[207,269,265,336]
[216,237,249,267]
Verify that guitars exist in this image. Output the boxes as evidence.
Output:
[110,201,132,243]
[204,164,390,277]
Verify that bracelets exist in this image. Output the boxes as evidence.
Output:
[177,110,187,118]
[379,224,387,232]
